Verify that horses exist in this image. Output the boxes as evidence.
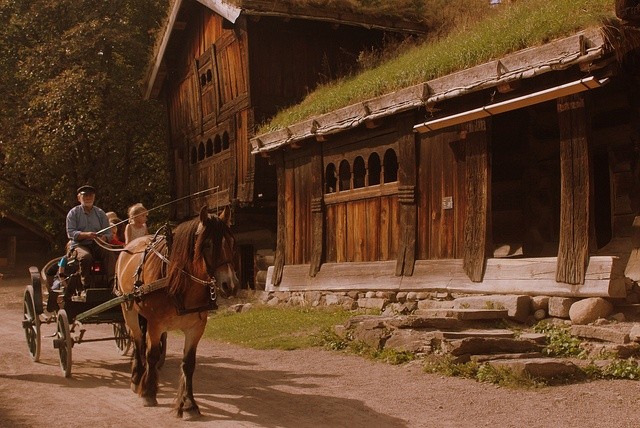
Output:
[115,204,241,418]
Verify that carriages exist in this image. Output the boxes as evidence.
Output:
[22,204,239,420]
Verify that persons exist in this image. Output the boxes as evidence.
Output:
[106,211,124,245]
[125,203,147,244]
[67,185,118,296]
[59,240,75,273]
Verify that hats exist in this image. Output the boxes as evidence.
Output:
[77,185,96,195]
